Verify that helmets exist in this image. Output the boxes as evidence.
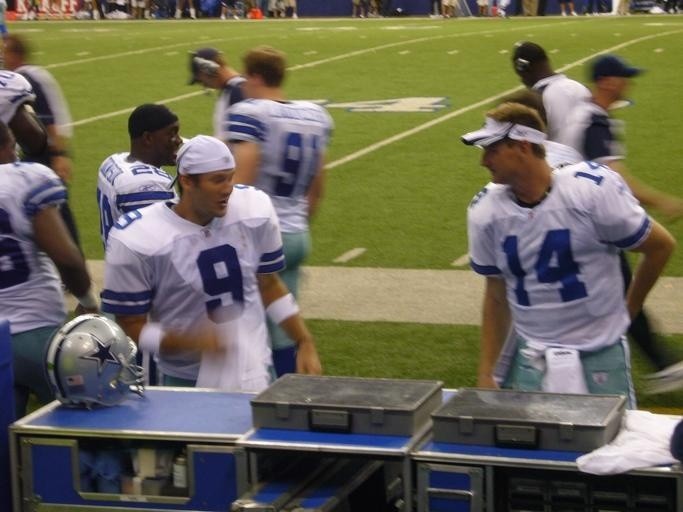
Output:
[45,311,147,409]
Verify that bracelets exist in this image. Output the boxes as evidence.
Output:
[49,150,67,157]
[73,287,99,309]
[295,332,313,347]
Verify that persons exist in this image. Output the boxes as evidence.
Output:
[560,53,682,378]
[188,47,247,130]
[497,91,584,169]
[458,100,678,407]
[96,103,181,251]
[216,43,336,374]
[1,0,682,22]
[0,32,84,290]
[0,120,99,417]
[99,135,324,392]
[0,38,50,157]
[512,42,591,140]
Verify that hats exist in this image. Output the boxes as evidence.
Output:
[189,48,227,87]
[165,135,237,189]
[460,119,546,149]
[588,54,642,82]
[124,104,180,139]
